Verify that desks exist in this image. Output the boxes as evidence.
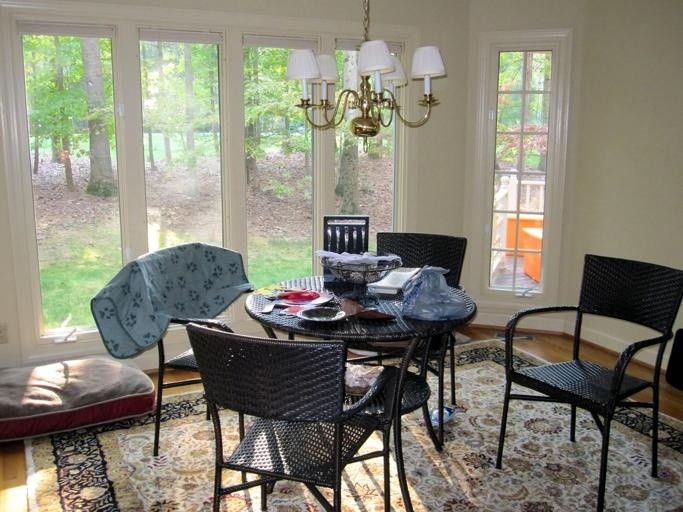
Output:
[665,324,683,397]
[241,274,477,512]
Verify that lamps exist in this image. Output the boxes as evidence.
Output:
[274,0,449,154]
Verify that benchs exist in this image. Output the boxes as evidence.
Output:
[522,226,543,282]
[500,211,543,257]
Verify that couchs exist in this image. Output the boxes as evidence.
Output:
[1,354,157,441]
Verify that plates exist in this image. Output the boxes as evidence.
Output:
[295,306,345,324]
[273,292,333,308]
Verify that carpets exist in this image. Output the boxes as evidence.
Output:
[21,333,683,512]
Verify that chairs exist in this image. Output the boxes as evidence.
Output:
[137,243,280,459]
[345,229,471,444]
[322,216,369,274]
[176,318,401,512]
[491,249,683,512]
